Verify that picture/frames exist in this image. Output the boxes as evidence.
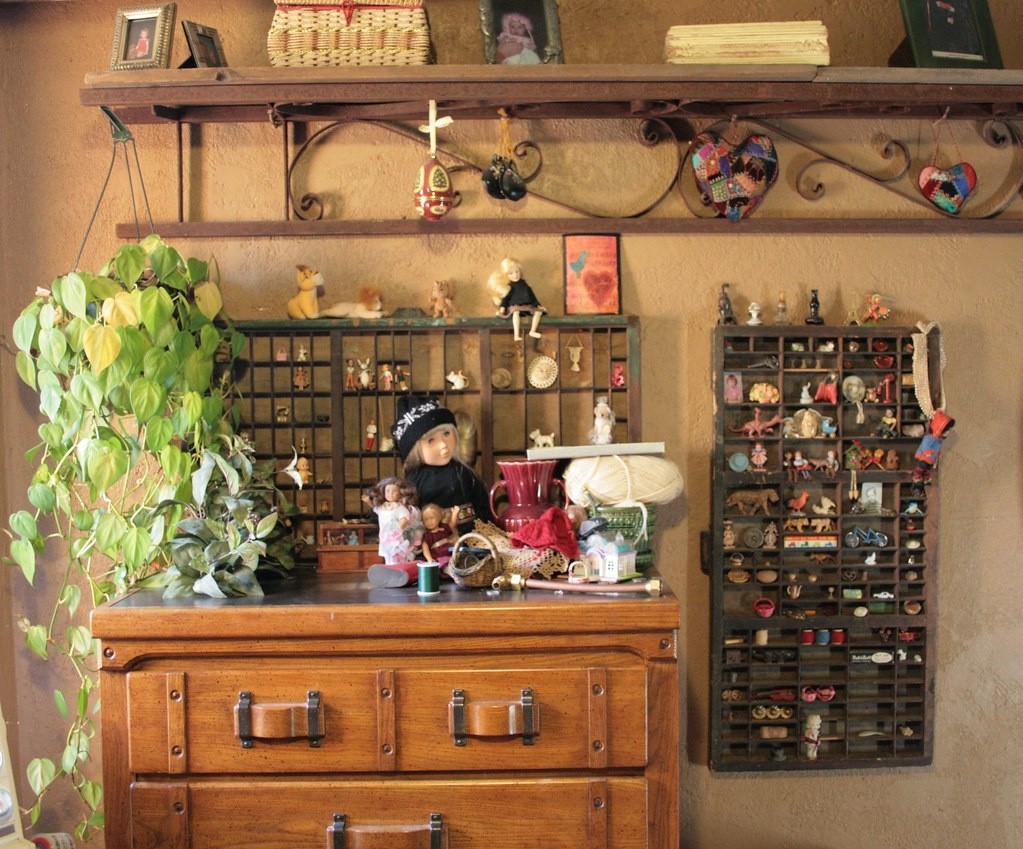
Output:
[478,1,564,64]
[183,21,231,72]
[112,3,178,72]
[890,0,1004,72]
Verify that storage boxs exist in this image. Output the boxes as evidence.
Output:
[263,1,439,68]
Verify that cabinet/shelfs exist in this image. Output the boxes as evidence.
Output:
[171,314,642,572]
[89,572,679,849]
[709,323,938,771]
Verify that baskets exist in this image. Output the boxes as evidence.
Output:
[589,504,657,565]
[267,0,432,67]
[449,534,503,588]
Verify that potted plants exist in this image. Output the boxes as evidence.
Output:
[14,237,292,835]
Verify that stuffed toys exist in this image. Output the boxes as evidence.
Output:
[529,429,555,447]
[356,355,376,390]
[287,264,455,319]
[586,396,616,445]
[445,370,469,390]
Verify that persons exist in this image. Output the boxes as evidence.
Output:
[496,35,523,65]
[723,293,896,760]
[296,457,312,483]
[348,531,359,545]
[395,365,410,391]
[136,27,150,60]
[365,418,376,450]
[324,529,334,545]
[421,503,461,578]
[379,364,393,391]
[362,478,415,565]
[391,394,497,537]
[395,481,425,554]
[496,12,541,65]
[346,358,356,391]
[294,367,310,390]
[336,533,347,545]
[487,257,548,341]
